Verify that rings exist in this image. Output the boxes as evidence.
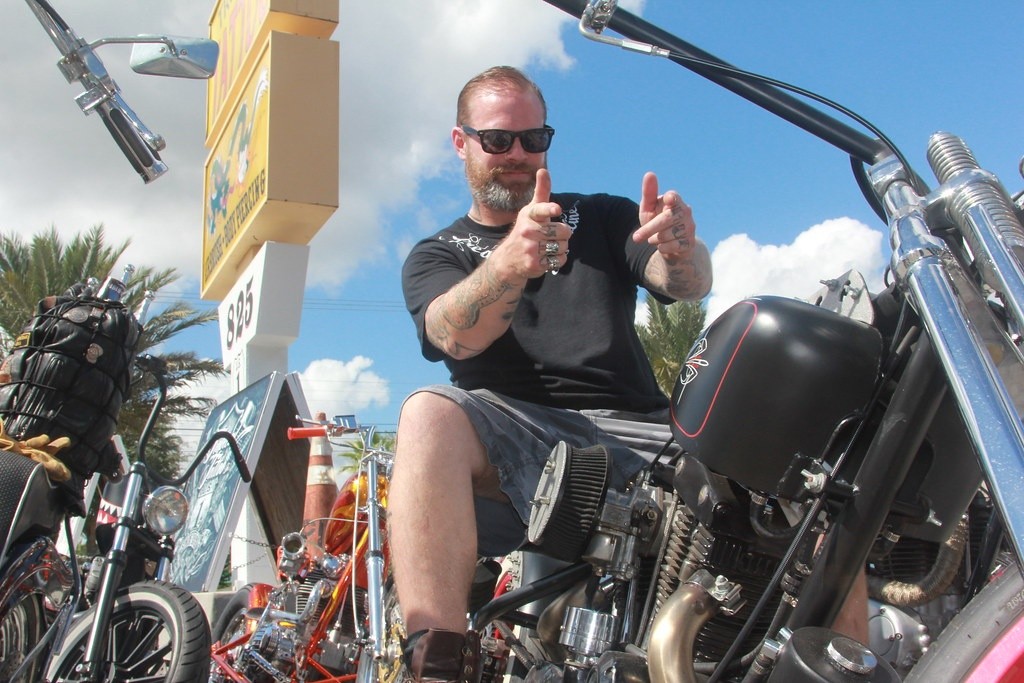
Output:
[548,256,559,272]
[545,241,559,256]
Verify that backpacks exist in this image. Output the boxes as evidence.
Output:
[0,280,143,516]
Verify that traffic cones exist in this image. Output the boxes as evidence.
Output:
[300,413,341,573]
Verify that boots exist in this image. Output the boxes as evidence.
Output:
[404,626,484,683]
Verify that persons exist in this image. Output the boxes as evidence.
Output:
[386,66,869,683]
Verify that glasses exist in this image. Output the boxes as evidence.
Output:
[461,124,554,154]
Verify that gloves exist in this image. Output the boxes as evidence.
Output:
[0,419,73,481]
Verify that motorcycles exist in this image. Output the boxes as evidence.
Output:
[0,1,1024,683]
